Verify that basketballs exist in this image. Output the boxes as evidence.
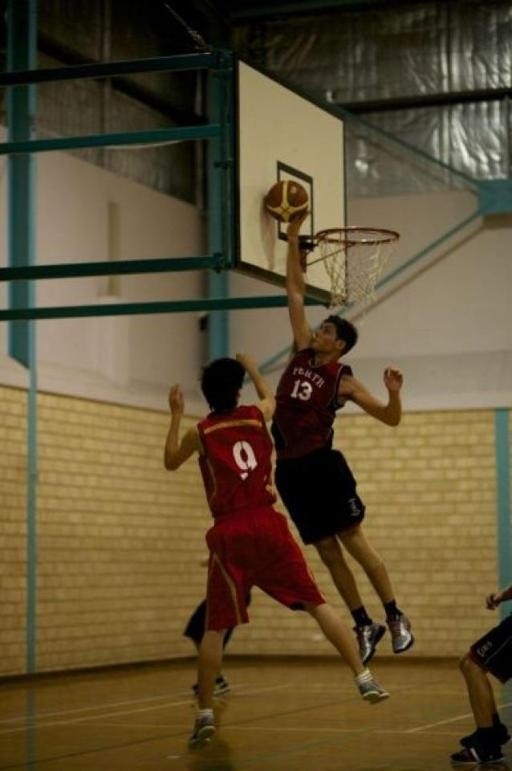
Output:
[266,179,308,222]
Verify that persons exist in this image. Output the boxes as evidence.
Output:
[180,578,252,697]
[448,581,511,766]
[159,350,389,750]
[270,208,415,668]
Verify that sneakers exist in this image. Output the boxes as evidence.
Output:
[386,610,413,653]
[359,678,389,703]
[449,744,505,764]
[193,675,229,695]
[354,620,386,664]
[459,722,510,747]
[190,717,215,748]
